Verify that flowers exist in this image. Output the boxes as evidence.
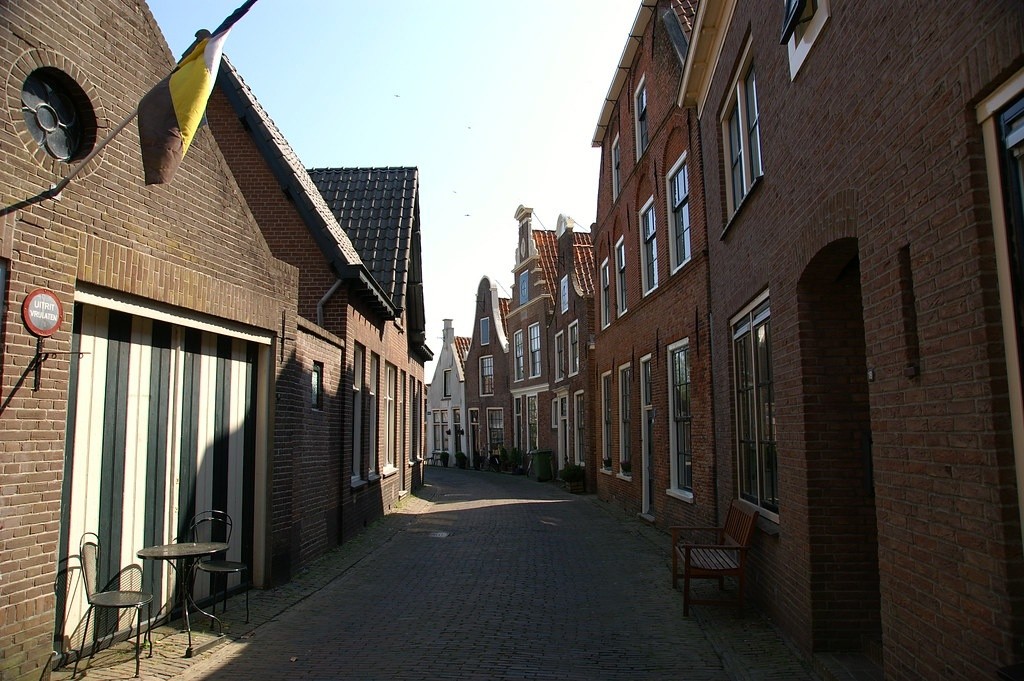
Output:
[619,460,629,465]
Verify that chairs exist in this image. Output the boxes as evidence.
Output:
[185,510,249,631]
[71,532,154,679]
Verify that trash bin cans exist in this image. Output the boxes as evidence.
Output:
[529,449,553,482]
[433,451,449,467]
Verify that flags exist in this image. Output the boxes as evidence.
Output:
[137,29,229,185]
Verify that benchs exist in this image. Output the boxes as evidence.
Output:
[669,497,760,621]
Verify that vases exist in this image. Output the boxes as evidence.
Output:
[621,464,631,472]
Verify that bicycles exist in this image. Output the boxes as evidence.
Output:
[474,443,500,473]
[527,451,534,478]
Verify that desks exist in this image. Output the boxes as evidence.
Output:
[137,542,230,658]
